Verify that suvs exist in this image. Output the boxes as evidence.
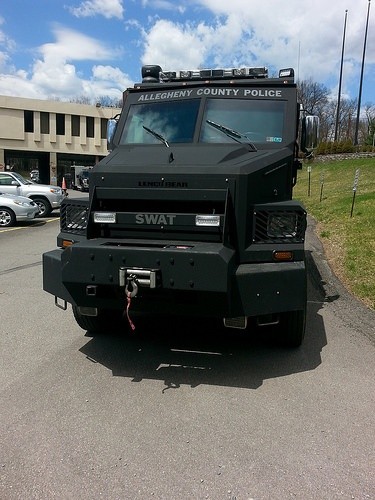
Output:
[42,63,320,350]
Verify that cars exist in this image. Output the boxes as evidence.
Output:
[0,171,66,217]
[77,170,90,192]
[0,193,41,227]
